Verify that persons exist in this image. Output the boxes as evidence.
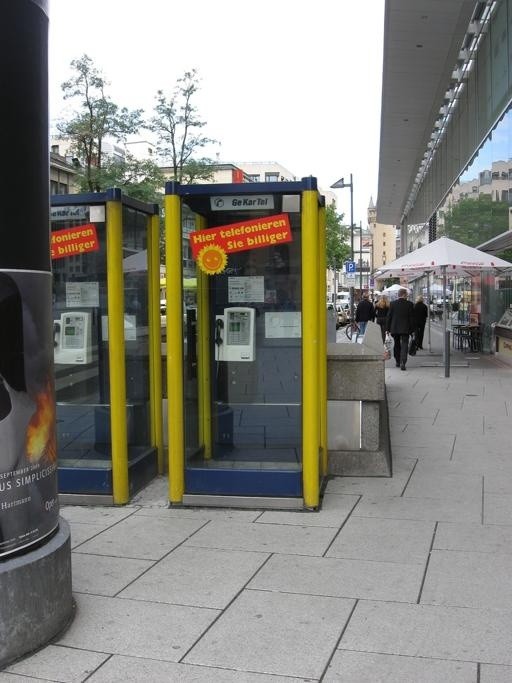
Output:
[356,288,427,369]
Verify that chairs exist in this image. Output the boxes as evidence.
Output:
[452,323,484,353]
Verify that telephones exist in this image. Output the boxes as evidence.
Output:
[53,311,94,366]
[214,306,258,363]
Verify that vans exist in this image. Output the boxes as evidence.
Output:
[335,289,351,303]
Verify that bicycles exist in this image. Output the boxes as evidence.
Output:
[343,305,362,340]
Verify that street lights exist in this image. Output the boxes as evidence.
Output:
[329,171,357,288]
[346,220,364,289]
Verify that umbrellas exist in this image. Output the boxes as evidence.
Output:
[368,233,512,362]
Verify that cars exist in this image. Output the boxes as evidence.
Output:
[325,300,358,331]
[423,286,462,314]
[158,299,187,341]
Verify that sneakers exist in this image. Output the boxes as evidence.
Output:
[396,358,400,368]
[401,361,406,370]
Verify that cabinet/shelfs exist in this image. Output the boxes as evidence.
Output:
[489,307,512,366]
